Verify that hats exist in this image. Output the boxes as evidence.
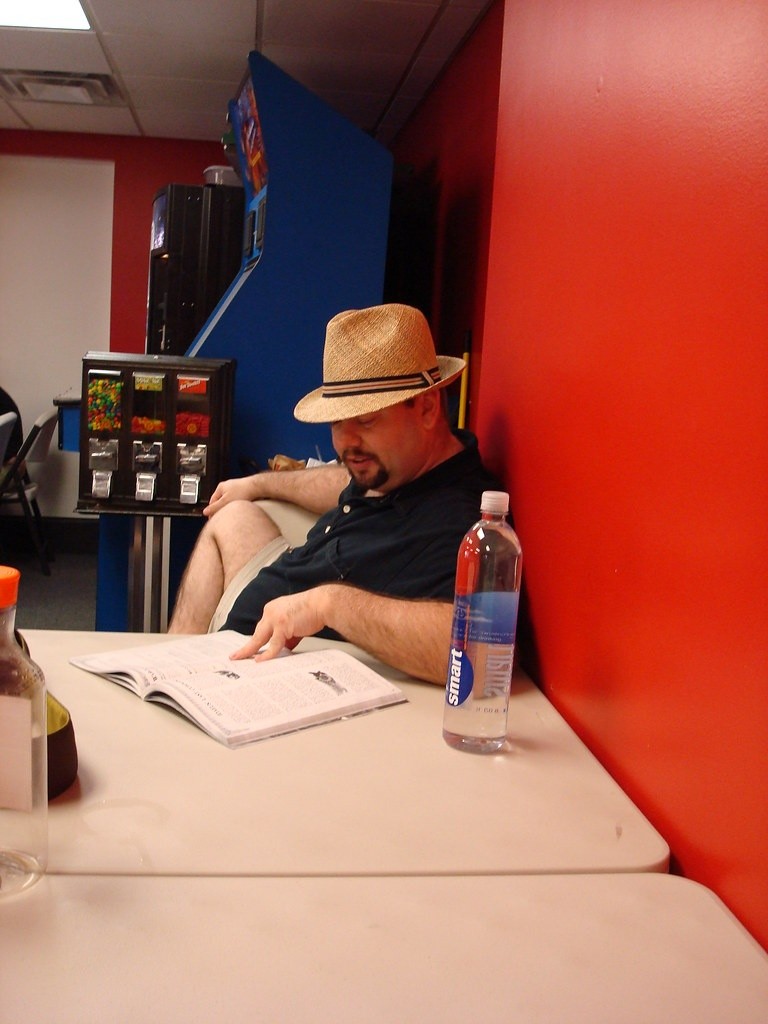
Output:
[293,301,468,424]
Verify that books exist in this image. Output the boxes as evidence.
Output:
[67,629,409,749]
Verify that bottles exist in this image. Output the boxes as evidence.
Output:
[0,563,51,891]
[437,490,525,748]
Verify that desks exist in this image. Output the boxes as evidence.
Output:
[0,629,668,873]
[0,875,764,1023]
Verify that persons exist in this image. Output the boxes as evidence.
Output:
[0,386,26,492]
[166,303,521,689]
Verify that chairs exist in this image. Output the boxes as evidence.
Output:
[0,412,17,466]
[0,407,59,574]
